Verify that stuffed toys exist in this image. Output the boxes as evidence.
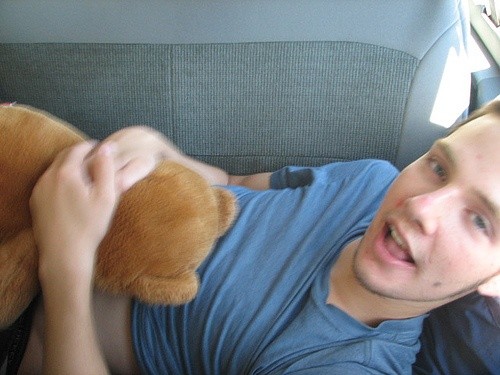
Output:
[0,103,238,329]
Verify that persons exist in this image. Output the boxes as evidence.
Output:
[0,96,500,375]
